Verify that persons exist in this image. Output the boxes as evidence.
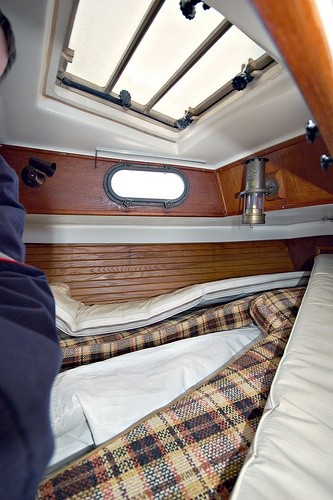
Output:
[0,5,64,500]
